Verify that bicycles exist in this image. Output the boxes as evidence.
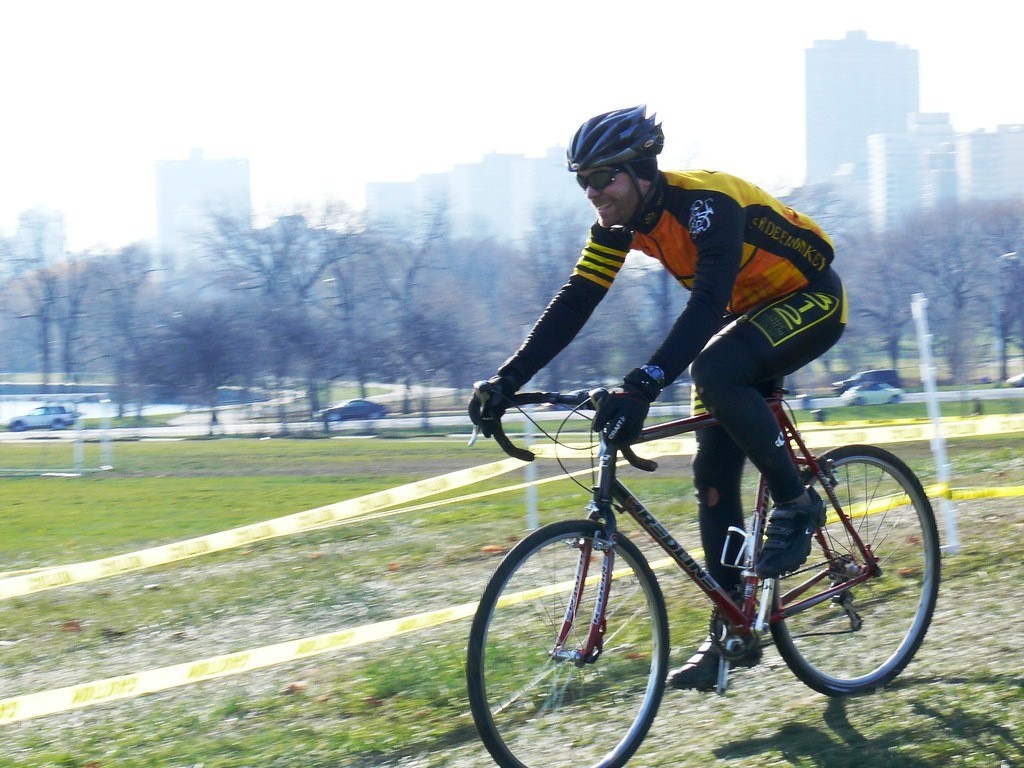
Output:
[465,357,941,768]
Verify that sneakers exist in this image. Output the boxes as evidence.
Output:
[753,485,827,580]
[666,634,762,690]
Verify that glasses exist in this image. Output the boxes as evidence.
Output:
[576,165,629,190]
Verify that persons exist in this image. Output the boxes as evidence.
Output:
[468,104,848,690]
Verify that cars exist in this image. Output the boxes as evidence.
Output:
[841,381,904,408]
[542,388,598,411]
[312,398,388,424]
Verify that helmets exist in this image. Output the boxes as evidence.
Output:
[566,104,664,172]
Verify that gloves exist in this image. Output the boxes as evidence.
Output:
[467,376,516,438]
[591,367,661,451]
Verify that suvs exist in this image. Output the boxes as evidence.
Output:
[3,401,79,431]
[830,368,900,395]
